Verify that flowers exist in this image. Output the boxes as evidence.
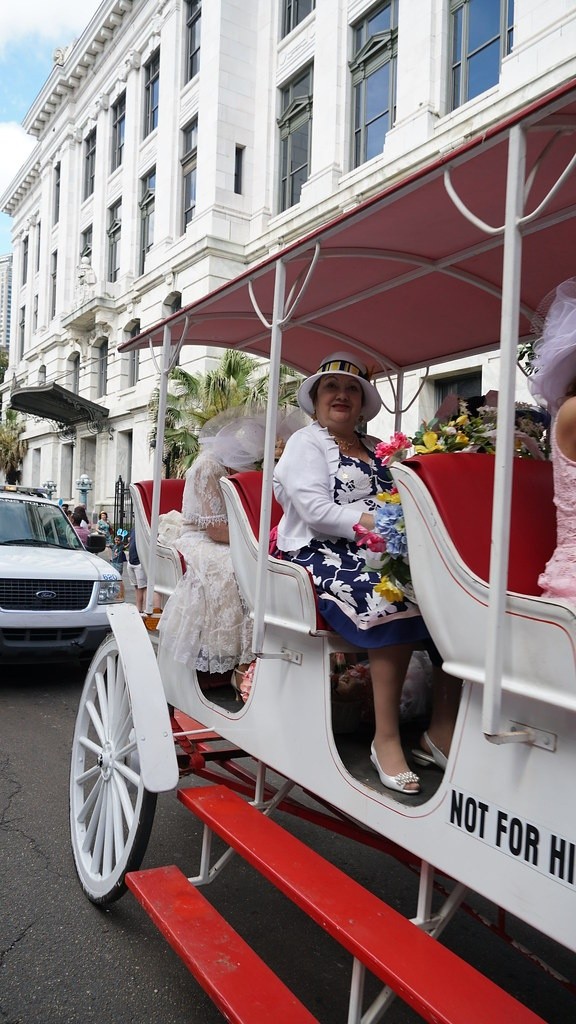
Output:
[352,402,550,605]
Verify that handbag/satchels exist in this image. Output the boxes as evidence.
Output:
[107,522,114,535]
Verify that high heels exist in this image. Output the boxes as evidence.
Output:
[368,740,420,794]
[411,731,448,771]
[231,669,247,700]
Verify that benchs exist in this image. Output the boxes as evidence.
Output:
[126,479,186,598]
[218,469,341,640]
[387,451,576,714]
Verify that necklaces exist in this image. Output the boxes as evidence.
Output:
[331,433,356,451]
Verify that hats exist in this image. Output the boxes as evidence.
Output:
[100,510,107,514]
[528,277,576,418]
[199,406,307,473]
[297,351,381,423]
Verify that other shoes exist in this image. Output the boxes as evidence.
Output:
[140,612,146,617]
[113,597,124,600]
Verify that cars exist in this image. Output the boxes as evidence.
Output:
[0,492,125,674]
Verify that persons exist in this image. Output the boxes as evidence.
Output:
[106,536,129,575]
[526,272,575,607]
[127,526,147,612]
[62,504,91,547]
[95,510,113,544]
[156,399,311,701]
[272,352,462,796]
